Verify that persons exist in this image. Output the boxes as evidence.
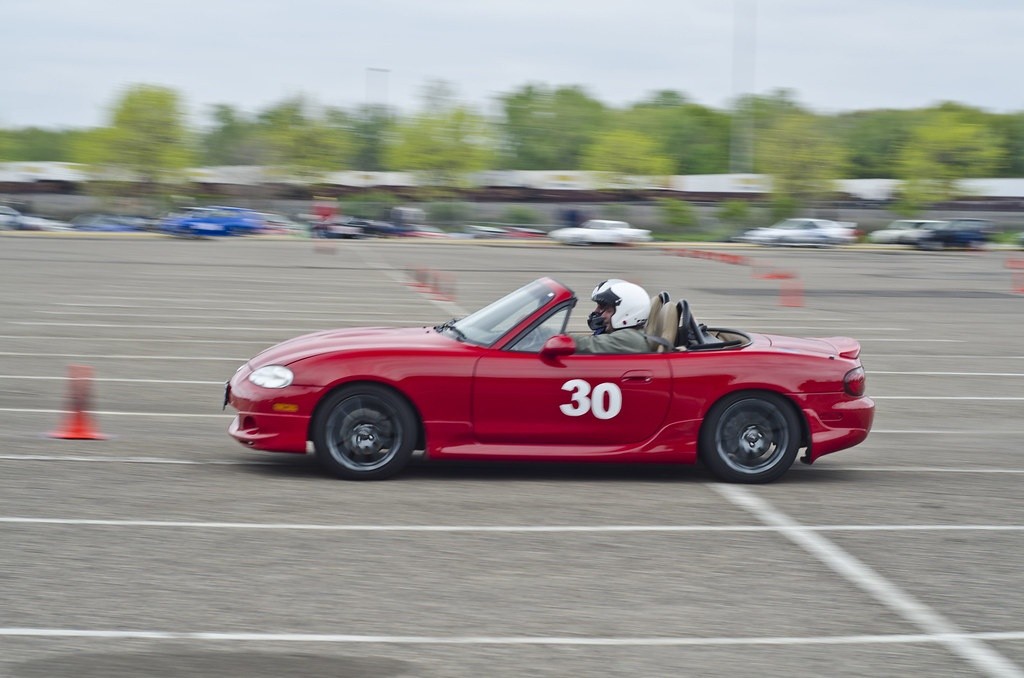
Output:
[524,279,650,354]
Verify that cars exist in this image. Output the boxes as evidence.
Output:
[867,217,990,251]
[547,219,653,246]
[740,218,859,248]
[0,202,548,235]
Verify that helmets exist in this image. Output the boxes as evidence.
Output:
[587,279,627,330]
[593,283,650,330]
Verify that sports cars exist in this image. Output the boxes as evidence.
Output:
[222,275,876,481]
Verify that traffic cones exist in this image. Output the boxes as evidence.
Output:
[45,360,112,443]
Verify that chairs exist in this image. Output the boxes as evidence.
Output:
[652,300,690,353]
[645,291,670,348]
